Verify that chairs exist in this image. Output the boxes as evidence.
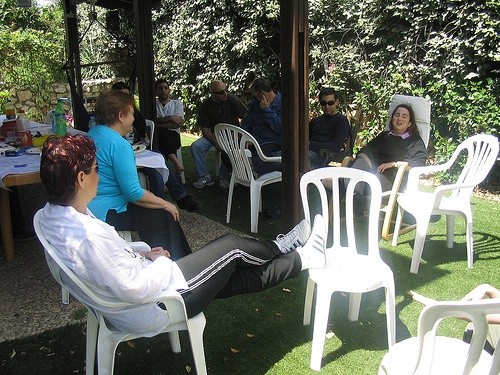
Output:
[34,95,500,375]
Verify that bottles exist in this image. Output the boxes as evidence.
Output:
[88,117,96,129]
[55,100,66,136]
[51,111,55,133]
[17,110,31,146]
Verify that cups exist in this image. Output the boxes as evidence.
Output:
[6,130,15,141]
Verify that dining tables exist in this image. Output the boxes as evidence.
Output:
[0,114,169,262]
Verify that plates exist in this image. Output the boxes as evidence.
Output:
[132,145,146,154]
[25,147,41,154]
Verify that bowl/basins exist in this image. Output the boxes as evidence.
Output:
[31,134,48,147]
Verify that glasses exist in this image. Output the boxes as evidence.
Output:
[251,93,260,99]
[212,86,228,95]
[320,100,337,106]
[84,161,99,174]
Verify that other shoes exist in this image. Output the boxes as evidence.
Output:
[242,157,257,180]
[176,196,199,212]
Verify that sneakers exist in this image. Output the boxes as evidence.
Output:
[297,214,326,269]
[274,219,311,255]
[219,179,229,191]
[193,175,215,188]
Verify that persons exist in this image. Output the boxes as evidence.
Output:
[192,80,249,189]
[111,81,201,213]
[86,89,193,261]
[221,79,426,218]
[155,79,185,174]
[38,132,326,340]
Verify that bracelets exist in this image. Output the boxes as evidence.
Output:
[395,161,397,168]
